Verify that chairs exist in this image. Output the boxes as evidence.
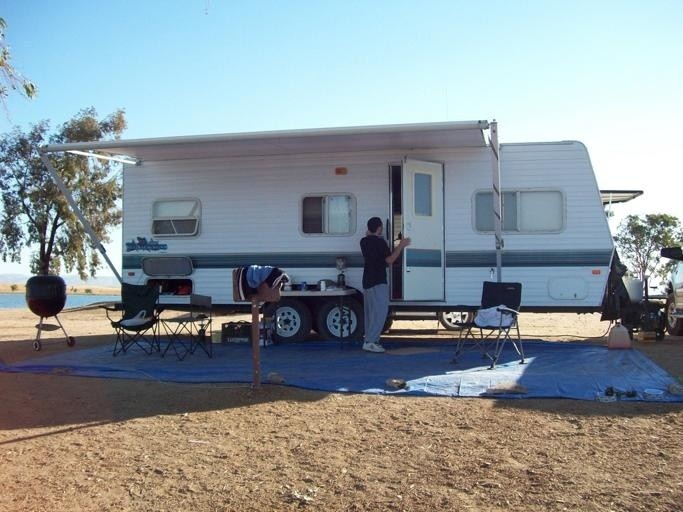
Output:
[98,282,213,361]
[453,281,525,369]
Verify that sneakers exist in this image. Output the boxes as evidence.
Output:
[362,342,386,353]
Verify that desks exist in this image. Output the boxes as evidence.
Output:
[262,288,358,351]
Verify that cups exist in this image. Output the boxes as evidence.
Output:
[301,281,307,291]
[284,283,292,291]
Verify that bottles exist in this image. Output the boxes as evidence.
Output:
[320,280,326,291]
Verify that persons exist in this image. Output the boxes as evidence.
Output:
[359,217,410,352]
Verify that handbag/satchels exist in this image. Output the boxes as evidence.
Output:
[120,310,152,327]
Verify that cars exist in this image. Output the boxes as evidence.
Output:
[661,247,683,335]
